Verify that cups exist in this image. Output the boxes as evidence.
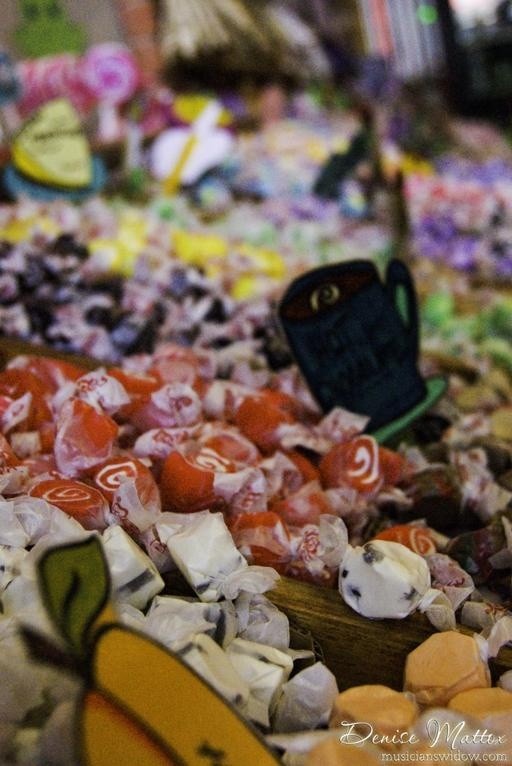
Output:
[278,257,422,435]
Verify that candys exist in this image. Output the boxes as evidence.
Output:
[0,44,512,766]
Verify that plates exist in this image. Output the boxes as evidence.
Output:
[366,377,448,451]
